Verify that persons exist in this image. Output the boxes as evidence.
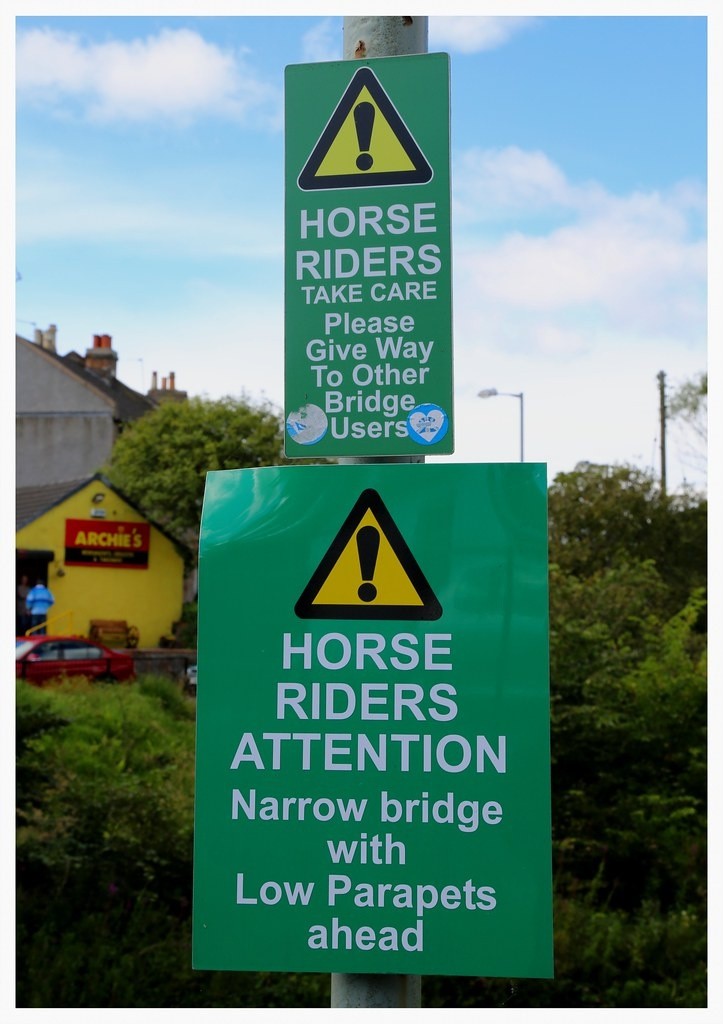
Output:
[15,575,54,635]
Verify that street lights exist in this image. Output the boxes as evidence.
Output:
[477,389,524,461]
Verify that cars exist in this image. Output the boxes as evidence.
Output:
[15,637,134,689]
[185,663,197,698]
